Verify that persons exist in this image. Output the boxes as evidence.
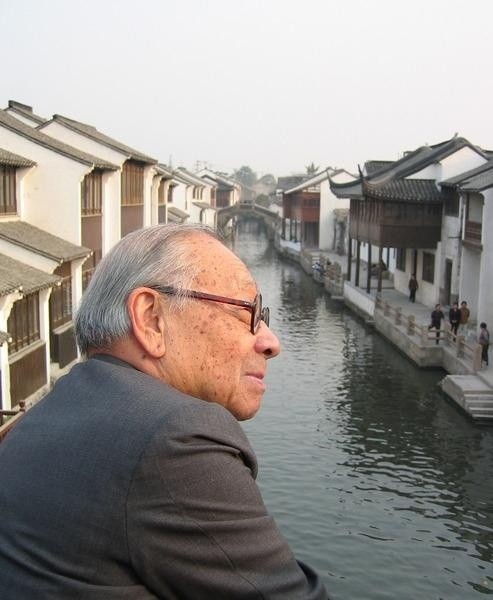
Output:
[407,273,418,304]
[428,304,444,345]
[477,322,489,366]
[459,301,469,340]
[0,220,327,597]
[448,302,461,343]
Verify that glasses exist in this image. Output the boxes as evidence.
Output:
[147,282,273,334]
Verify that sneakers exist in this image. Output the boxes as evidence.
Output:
[408,299,488,369]
